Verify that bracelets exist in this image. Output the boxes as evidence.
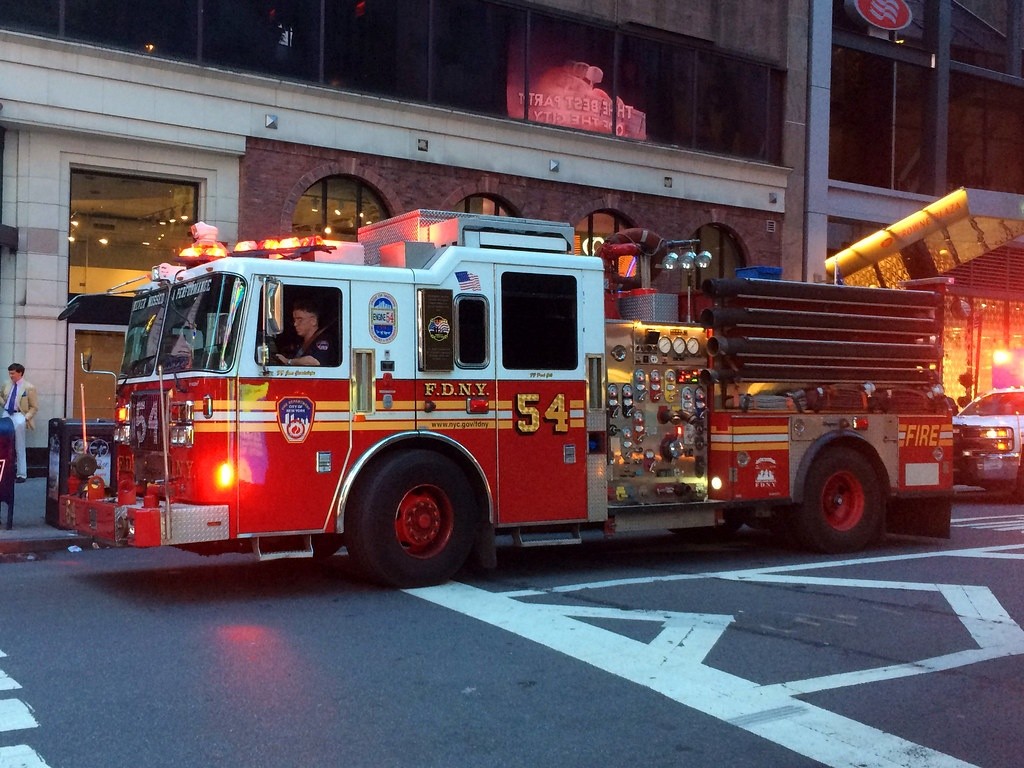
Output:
[287,358,292,366]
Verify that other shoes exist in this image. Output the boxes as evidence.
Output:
[16,477,25,483]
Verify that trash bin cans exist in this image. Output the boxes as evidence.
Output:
[0,417,17,532]
[45,417,118,531]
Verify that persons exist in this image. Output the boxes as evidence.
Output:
[275,302,337,366]
[1,362,39,482]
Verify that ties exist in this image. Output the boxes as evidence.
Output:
[8,383,17,416]
